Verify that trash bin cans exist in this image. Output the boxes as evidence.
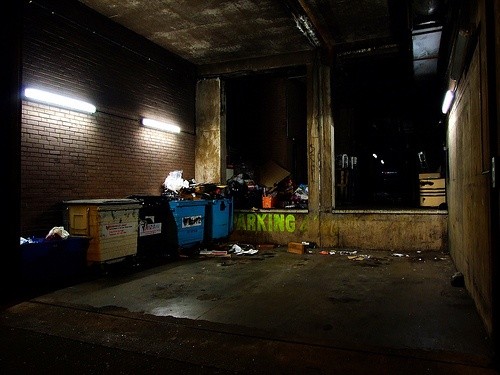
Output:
[125,191,177,266]
[62,198,143,281]
[17,228,90,296]
[194,186,236,246]
[158,189,209,255]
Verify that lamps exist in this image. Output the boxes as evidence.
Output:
[24,88,97,115]
[141,117,180,134]
[442,90,454,114]
